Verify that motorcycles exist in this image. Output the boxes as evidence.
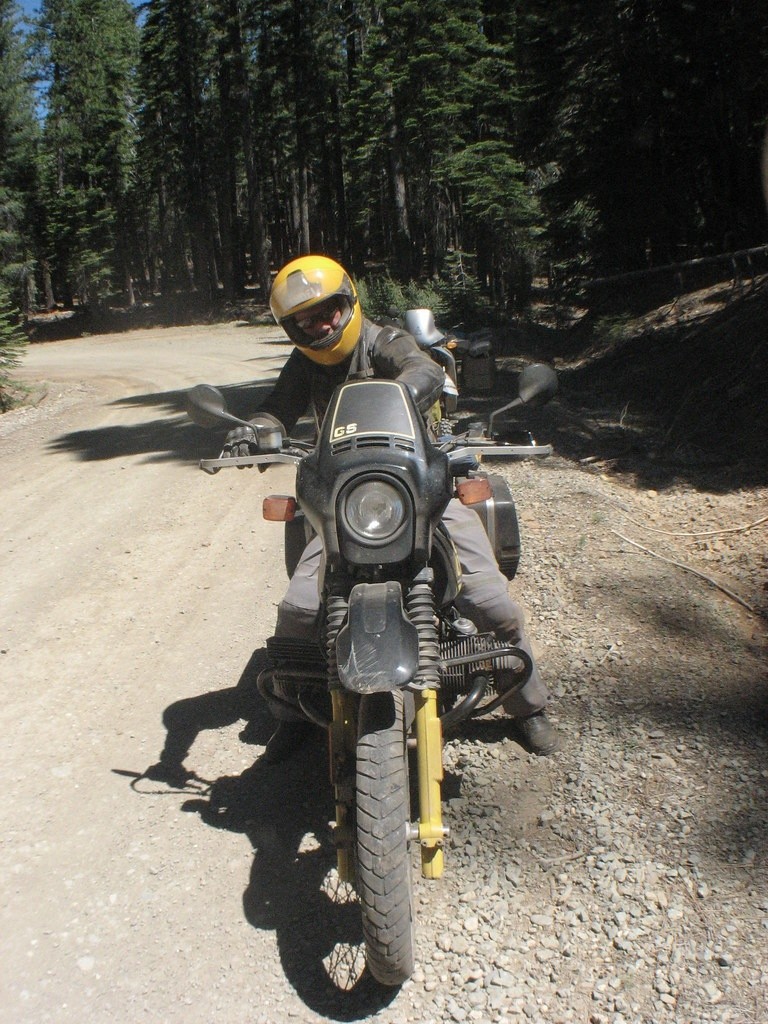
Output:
[182,307,562,986]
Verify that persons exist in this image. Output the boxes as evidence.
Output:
[224,254,561,765]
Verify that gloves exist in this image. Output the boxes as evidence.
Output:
[223,424,257,469]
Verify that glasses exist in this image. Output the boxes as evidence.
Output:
[295,301,339,330]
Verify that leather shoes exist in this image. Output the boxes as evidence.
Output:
[514,713,560,754]
[262,720,308,764]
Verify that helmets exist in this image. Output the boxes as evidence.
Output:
[268,255,362,367]
